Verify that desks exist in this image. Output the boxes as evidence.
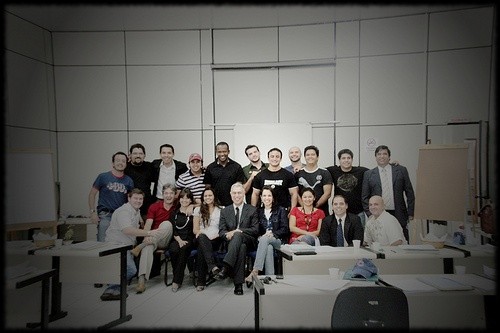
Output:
[252,245,496,329]
[6,240,134,330]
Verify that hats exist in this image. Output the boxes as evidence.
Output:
[189,153,202,162]
[351,258,378,279]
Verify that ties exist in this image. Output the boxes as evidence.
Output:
[336,218,345,248]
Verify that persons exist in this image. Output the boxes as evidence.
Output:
[294,145,333,217]
[362,145,415,244]
[128,143,188,202]
[363,195,409,246]
[88,151,134,288]
[193,186,222,291]
[319,194,364,247]
[202,142,248,208]
[293,149,399,231]
[130,183,201,293]
[241,145,270,205]
[174,153,207,200]
[251,148,298,218]
[213,183,259,295]
[101,189,163,301]
[168,188,196,292]
[289,188,325,246]
[123,144,152,188]
[245,185,291,282]
[285,146,305,174]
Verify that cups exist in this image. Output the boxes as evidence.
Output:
[55,239,62,249]
[328,268,339,279]
[455,266,466,278]
[352,240,360,248]
[373,242,379,251]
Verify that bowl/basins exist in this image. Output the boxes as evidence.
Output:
[431,241,444,249]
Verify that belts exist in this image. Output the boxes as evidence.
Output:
[100,208,114,213]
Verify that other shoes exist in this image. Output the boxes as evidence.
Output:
[212,266,219,274]
[136,284,145,294]
[245,271,258,282]
[100,287,129,301]
[196,285,204,291]
[171,282,180,293]
[130,246,141,257]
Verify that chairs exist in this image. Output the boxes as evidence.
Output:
[331,286,409,329]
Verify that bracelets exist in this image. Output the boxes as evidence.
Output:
[90,210,96,212]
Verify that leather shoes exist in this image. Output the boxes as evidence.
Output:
[212,269,227,281]
[234,283,243,295]
